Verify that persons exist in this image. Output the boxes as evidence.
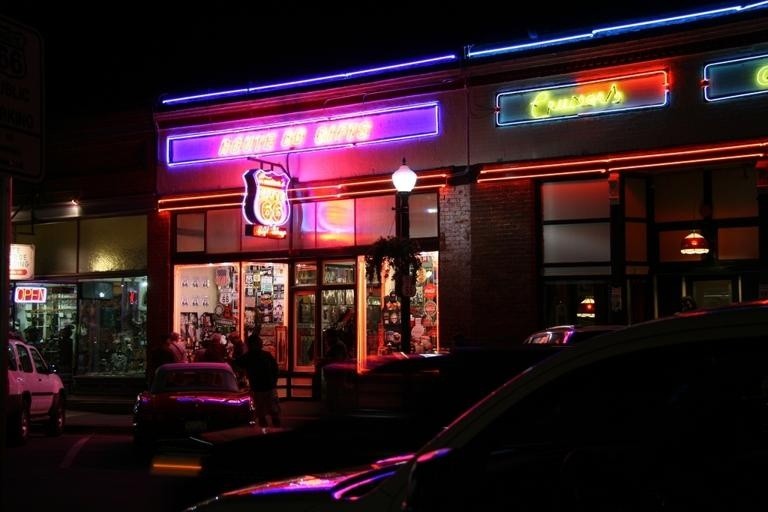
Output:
[58,328,73,383]
[308,328,349,402]
[152,330,248,392]
[236,333,281,430]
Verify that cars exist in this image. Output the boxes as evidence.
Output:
[38,334,66,369]
[132,299,768,512]
[136,361,259,427]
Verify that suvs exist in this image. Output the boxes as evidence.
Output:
[7,336,70,441]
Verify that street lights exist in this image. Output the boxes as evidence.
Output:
[390,156,418,357]
